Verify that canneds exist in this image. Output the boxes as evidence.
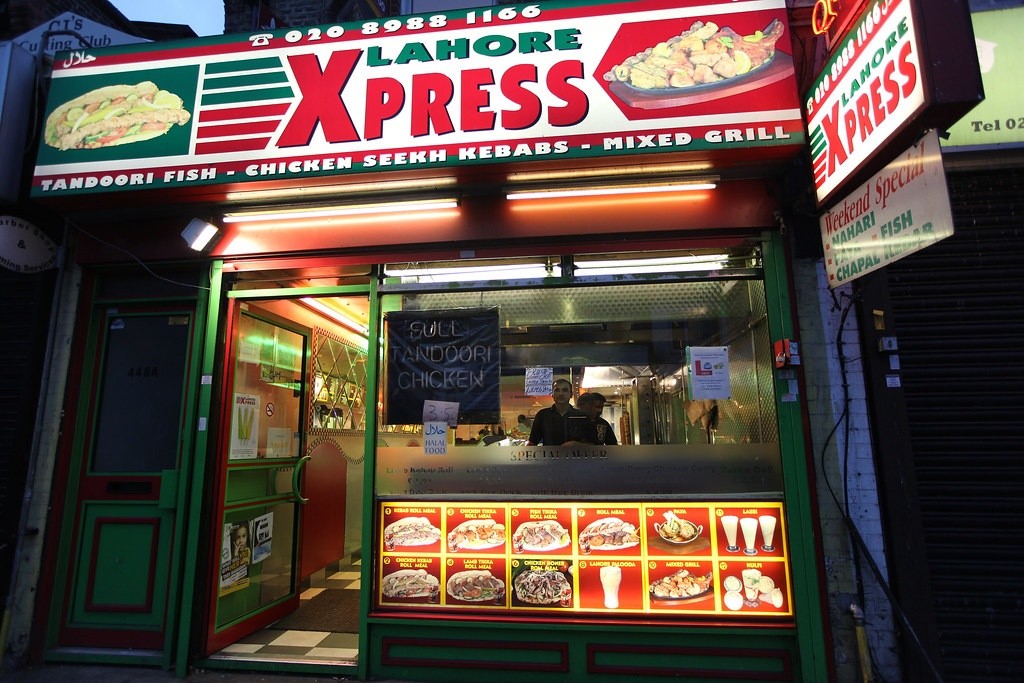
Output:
[385,531,395,551]
[448,532,459,552]
[513,534,524,553]
[560,586,572,607]
[493,586,504,605]
[428,584,438,603]
[579,534,591,555]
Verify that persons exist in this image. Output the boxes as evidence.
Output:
[232,522,251,566]
[527,379,618,446]
[479,426,504,439]
[509,414,531,436]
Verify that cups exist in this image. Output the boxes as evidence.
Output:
[599,566,623,609]
[739,517,759,555]
[720,514,741,552]
[758,515,777,553]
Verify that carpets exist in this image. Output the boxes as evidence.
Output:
[266,589,360,634]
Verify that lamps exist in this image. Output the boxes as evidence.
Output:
[181,219,219,254]
[384,258,562,278]
[574,253,731,269]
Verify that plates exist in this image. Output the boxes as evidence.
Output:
[759,576,775,593]
[448,518,504,550]
[514,520,570,552]
[649,582,712,600]
[382,569,440,598]
[621,50,777,94]
[579,516,640,550]
[653,517,704,546]
[723,576,743,592]
[384,516,441,546]
[724,591,744,610]
[448,569,505,601]
[512,566,573,606]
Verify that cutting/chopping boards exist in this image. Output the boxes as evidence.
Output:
[650,586,715,605]
[607,50,794,109]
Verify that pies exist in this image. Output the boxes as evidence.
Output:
[385,516,440,546]
[447,570,504,601]
[45,80,183,149]
[514,520,571,551]
[452,519,505,550]
[381,568,440,598]
[583,517,639,550]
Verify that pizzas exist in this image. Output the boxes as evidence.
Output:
[514,569,571,604]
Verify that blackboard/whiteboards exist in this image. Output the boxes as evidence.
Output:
[382,306,501,425]
